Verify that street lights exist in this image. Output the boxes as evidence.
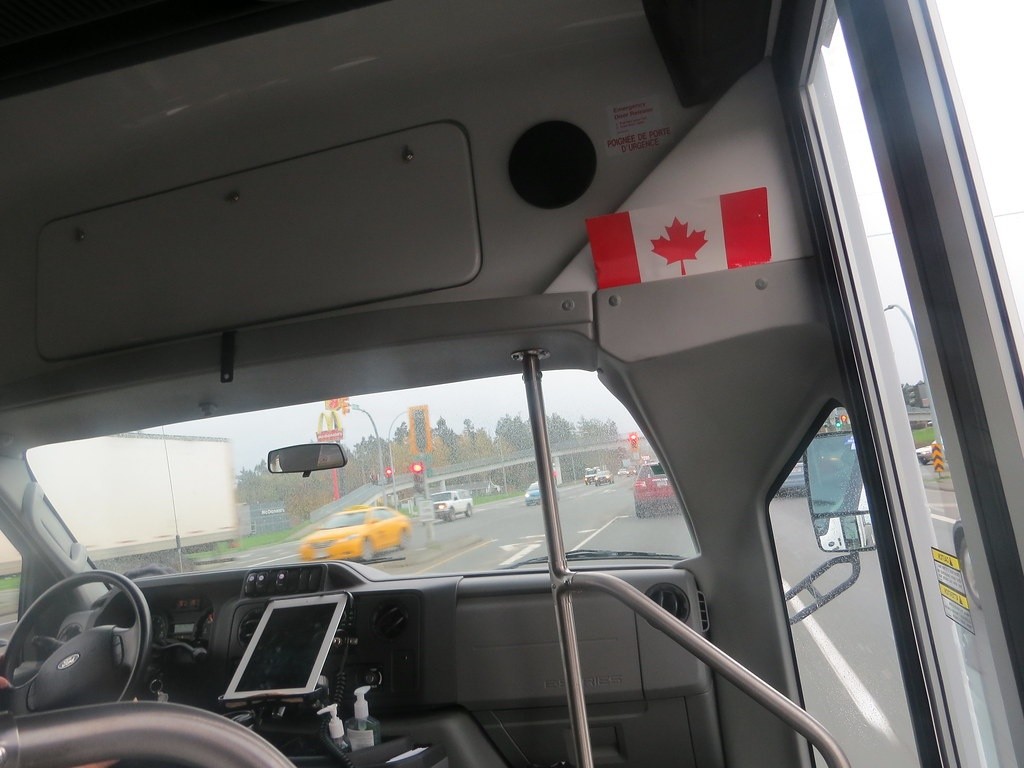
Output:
[883,304,945,452]
[350,403,387,507]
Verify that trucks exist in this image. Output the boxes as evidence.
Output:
[0,432,239,577]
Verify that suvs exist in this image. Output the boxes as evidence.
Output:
[585,467,600,485]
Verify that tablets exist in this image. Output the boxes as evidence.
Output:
[224,592,348,701]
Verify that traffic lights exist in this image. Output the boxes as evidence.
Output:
[835,416,841,428]
[412,461,425,493]
[386,468,392,478]
[841,415,846,422]
[630,434,638,448]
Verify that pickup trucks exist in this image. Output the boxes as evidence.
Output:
[431,490,474,522]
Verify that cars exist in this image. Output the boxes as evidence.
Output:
[774,456,806,499]
[633,461,680,519]
[525,481,560,506]
[916,440,937,465]
[617,463,640,477]
[299,505,412,563]
[594,470,614,486]
